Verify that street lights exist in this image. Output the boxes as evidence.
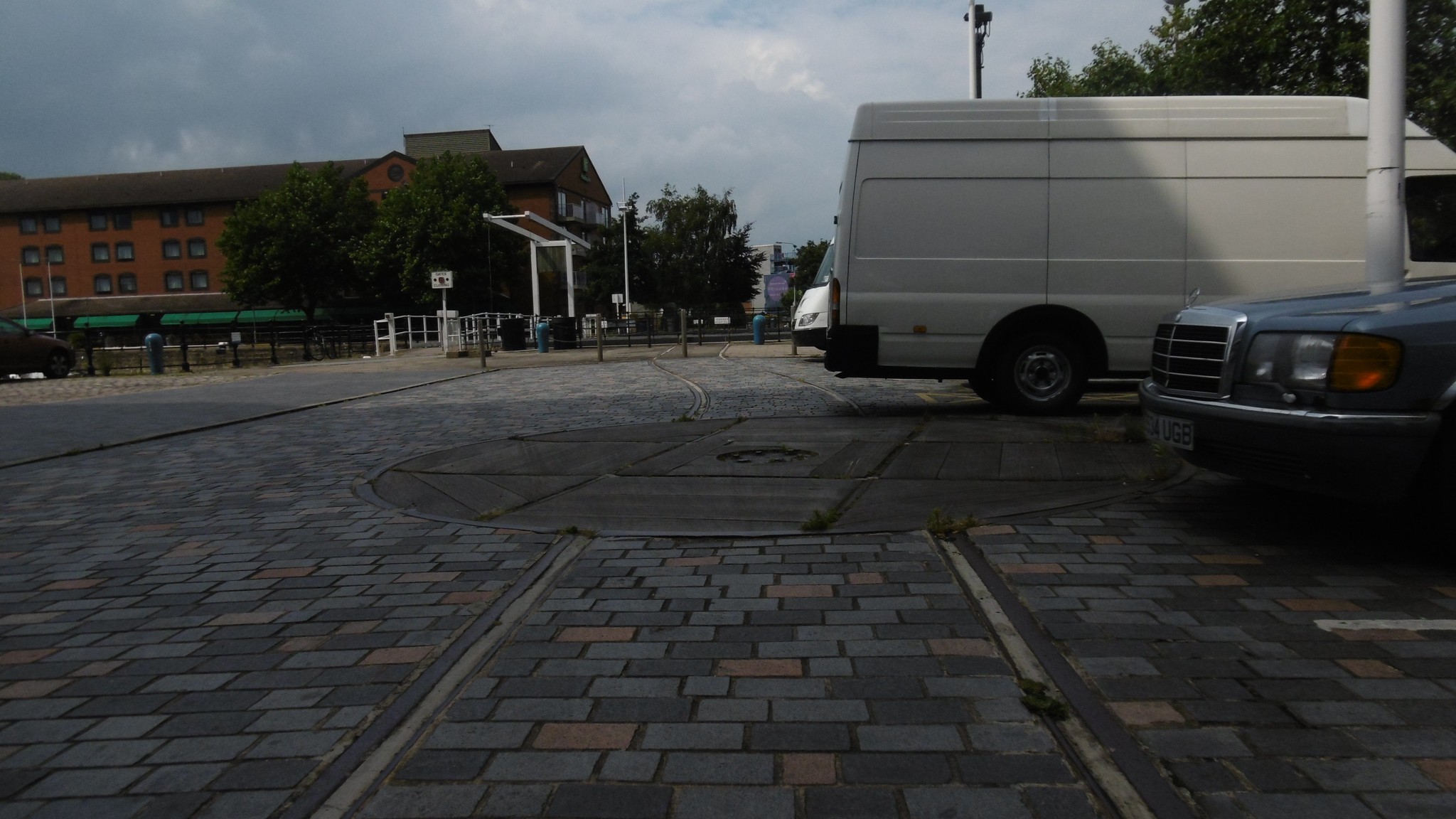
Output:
[775,241,797,308]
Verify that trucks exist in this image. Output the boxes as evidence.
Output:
[790,95,1456,415]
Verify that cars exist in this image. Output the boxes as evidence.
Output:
[1138,270,1455,540]
[0,317,77,380]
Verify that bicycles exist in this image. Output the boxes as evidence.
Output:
[303,325,336,360]
